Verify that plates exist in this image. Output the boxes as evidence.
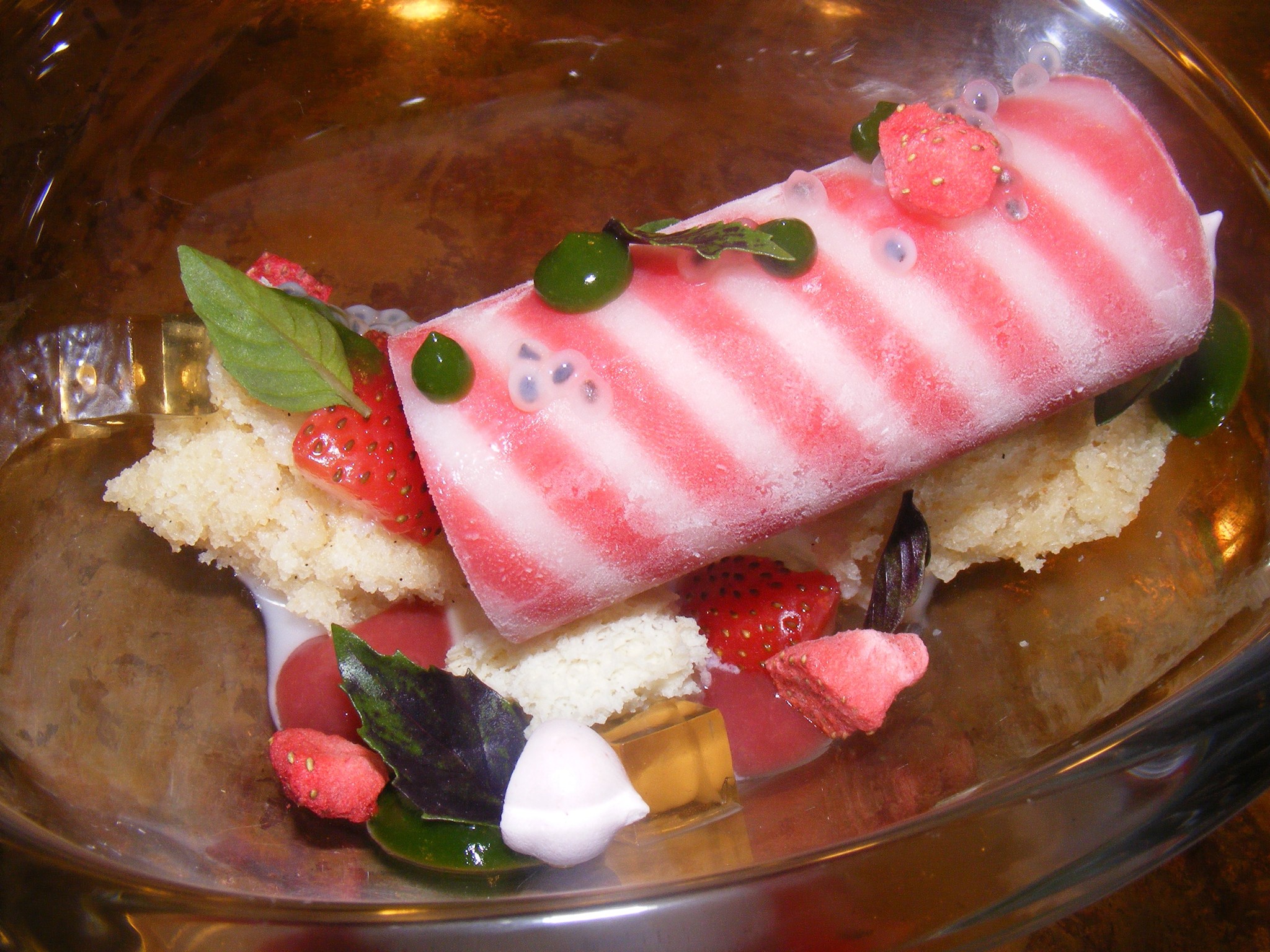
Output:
[1,0,1267,952]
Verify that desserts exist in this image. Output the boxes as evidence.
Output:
[95,48,1251,863]
